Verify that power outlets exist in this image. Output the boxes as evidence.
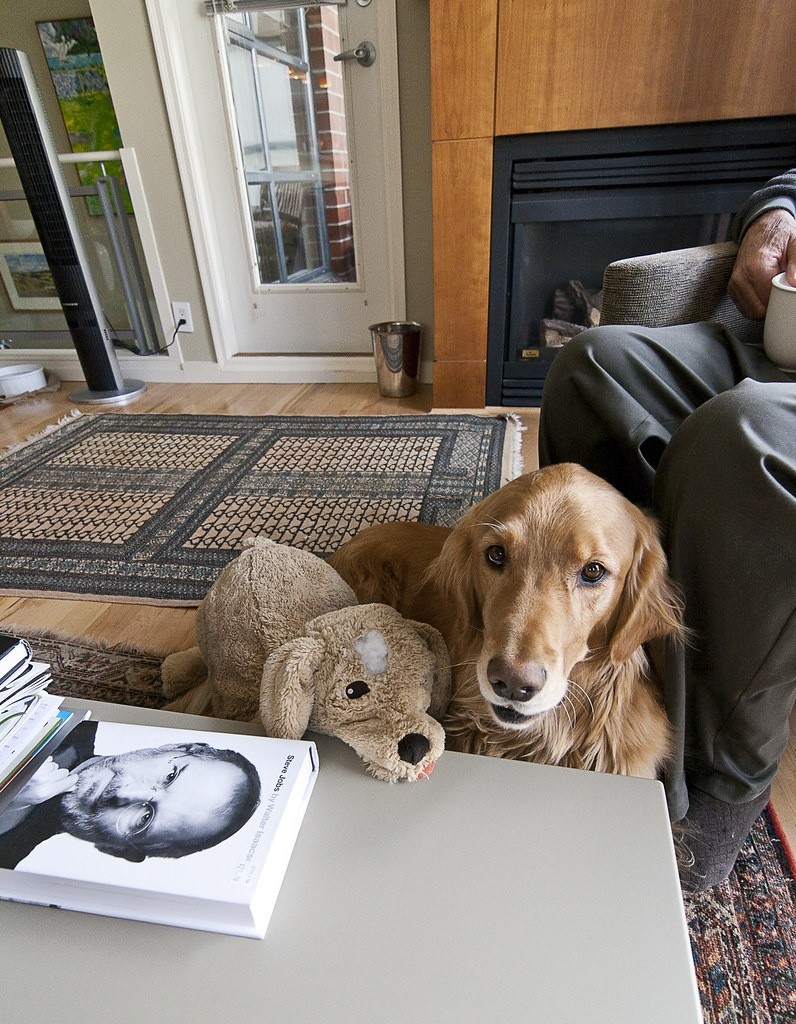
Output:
[172,302,194,333]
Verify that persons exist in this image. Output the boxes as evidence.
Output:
[538,168,796,895]
[0,720,261,870]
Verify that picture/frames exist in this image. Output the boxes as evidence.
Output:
[34,15,134,218]
[1,240,63,313]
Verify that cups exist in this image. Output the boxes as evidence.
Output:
[763,272,796,373]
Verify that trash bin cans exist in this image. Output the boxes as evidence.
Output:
[368,321,424,398]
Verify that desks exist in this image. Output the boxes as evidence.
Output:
[0,694,709,1024]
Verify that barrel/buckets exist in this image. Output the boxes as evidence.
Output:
[369,321,426,399]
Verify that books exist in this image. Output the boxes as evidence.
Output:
[0,634,74,792]
[0,719,321,939]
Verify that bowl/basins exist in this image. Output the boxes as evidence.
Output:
[0,365,47,398]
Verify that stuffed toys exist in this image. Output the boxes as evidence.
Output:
[159,535,453,782]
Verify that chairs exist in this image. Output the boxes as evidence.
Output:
[599,237,765,346]
[251,165,305,284]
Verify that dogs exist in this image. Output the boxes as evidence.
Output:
[133,461,699,778]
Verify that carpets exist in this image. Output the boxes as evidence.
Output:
[0,622,796,1024]
[0,407,527,608]
